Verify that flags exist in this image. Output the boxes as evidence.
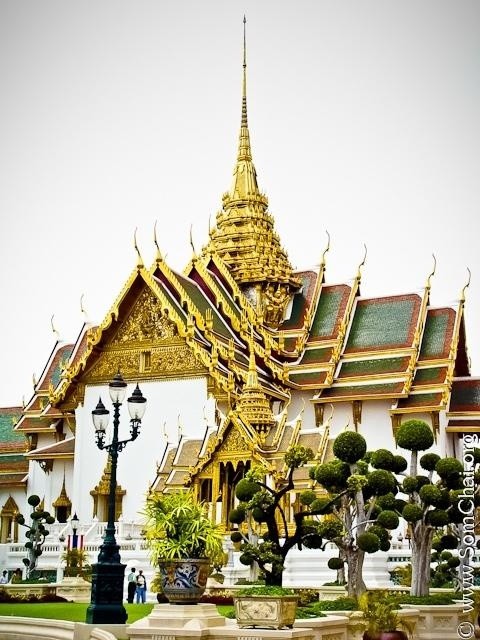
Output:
[67,535,84,568]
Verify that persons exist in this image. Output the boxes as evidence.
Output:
[0,570,9,583]
[126,567,138,603]
[11,568,22,583]
[136,570,148,604]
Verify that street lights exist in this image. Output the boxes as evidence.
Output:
[69,510,79,578]
[85,365,147,625]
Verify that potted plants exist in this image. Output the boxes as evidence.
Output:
[138,484,231,603]
[234,586,299,629]
[353,590,416,640]
